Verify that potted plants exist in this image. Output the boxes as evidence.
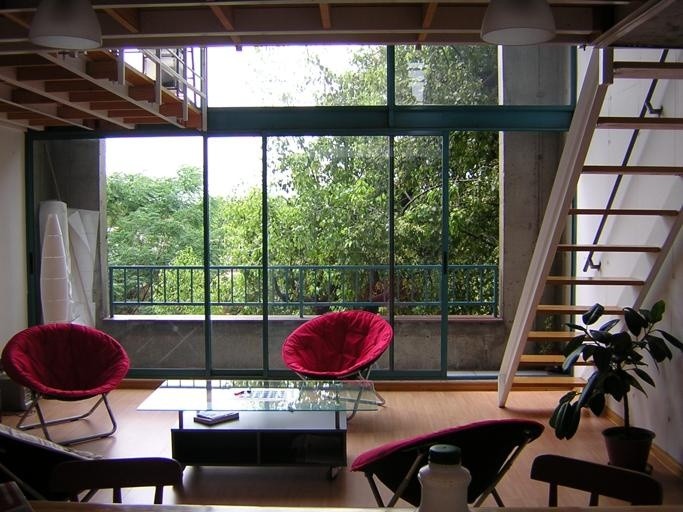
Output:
[547,300,683,476]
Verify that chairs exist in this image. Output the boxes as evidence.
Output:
[281,310,394,421]
[1,322,130,448]
[0,424,181,504]
[350,420,544,508]
[531,450,663,507]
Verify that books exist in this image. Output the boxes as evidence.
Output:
[194,409,239,424]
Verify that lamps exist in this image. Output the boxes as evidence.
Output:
[479,2,557,46]
[27,1,104,51]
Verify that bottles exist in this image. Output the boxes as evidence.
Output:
[415,445,474,511]
